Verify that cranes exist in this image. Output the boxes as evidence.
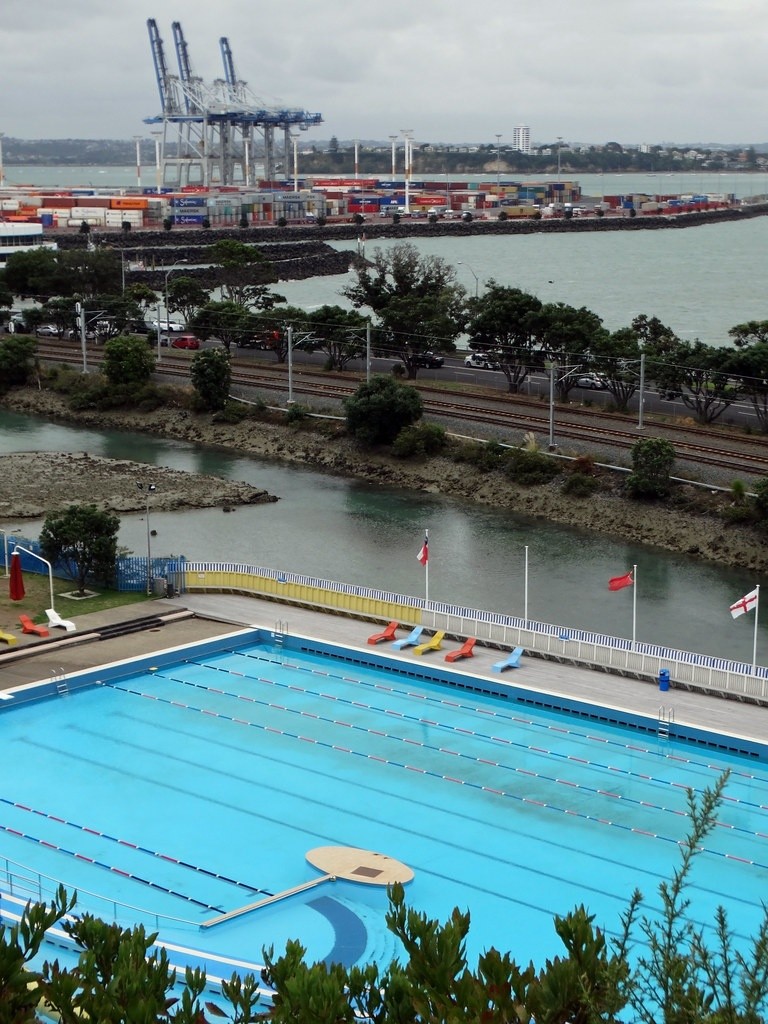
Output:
[142,16,242,122]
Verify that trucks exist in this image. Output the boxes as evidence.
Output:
[305,201,610,221]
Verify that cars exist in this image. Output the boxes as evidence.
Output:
[463,353,501,370]
[251,330,283,352]
[152,319,184,332]
[172,336,200,350]
[573,372,610,390]
[406,351,445,369]
[10,313,95,340]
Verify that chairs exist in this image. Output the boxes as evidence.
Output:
[368,621,399,644]
[45,609,76,631]
[414,630,445,654]
[392,626,424,649]
[446,638,477,662]
[492,647,524,672]
[0,630,18,645]
[18,614,48,636]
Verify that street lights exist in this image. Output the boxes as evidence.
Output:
[150,131,162,195]
[400,129,415,215]
[457,261,480,298]
[496,134,503,196]
[241,138,252,187]
[133,136,143,186]
[556,136,563,183]
[136,480,157,598]
[102,240,125,295]
[290,134,300,192]
[388,133,399,205]
[352,139,361,179]
[165,259,188,348]
